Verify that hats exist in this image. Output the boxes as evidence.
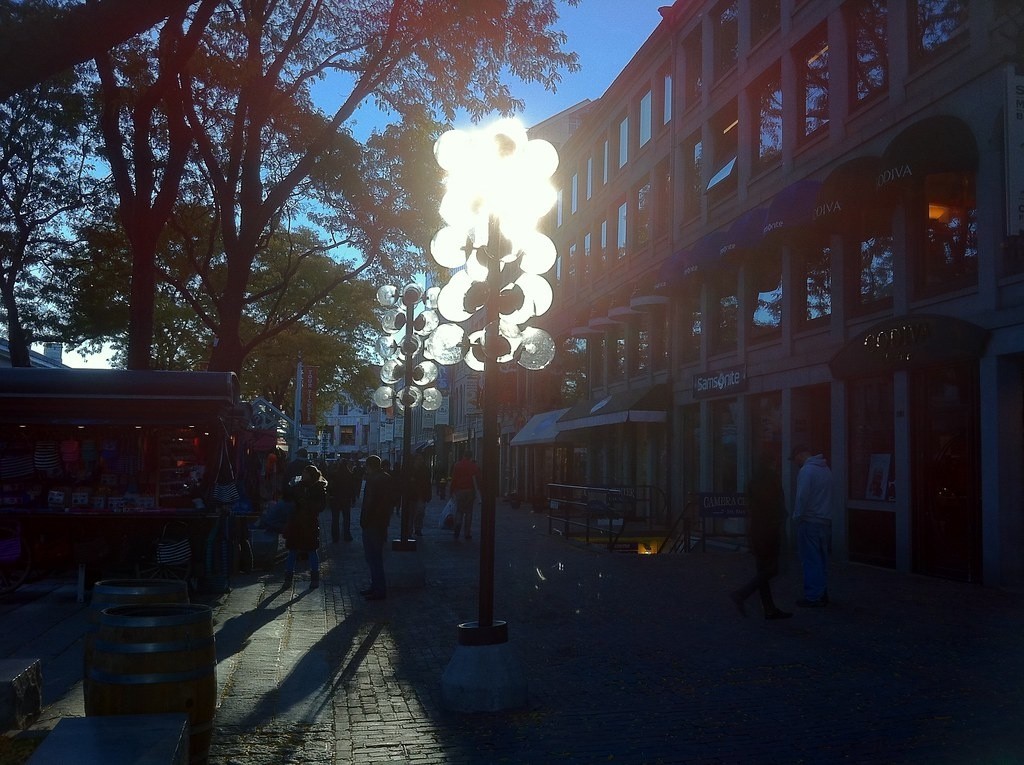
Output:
[788,443,808,462]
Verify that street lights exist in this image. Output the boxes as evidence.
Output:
[374,284,442,552]
[428,117,560,646]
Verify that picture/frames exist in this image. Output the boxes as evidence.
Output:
[864,450,893,500]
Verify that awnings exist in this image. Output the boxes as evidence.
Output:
[555,384,668,430]
[571,115,980,338]
[510,407,570,446]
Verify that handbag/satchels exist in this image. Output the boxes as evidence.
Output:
[59,441,81,461]
[0,454,36,480]
[33,441,61,470]
[157,541,192,571]
[439,498,457,530]
[213,482,241,503]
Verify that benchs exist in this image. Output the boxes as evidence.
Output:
[23,711,192,765]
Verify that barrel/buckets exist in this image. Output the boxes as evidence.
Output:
[84,579,218,765]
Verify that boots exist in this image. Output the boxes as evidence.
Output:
[283,573,293,587]
[310,570,320,588]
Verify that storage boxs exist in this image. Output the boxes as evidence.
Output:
[33,536,70,566]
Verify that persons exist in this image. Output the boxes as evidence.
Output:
[450,450,482,539]
[283,466,329,589]
[360,454,391,601]
[410,453,432,539]
[382,459,401,515]
[281,447,310,500]
[788,444,834,609]
[328,458,355,542]
[729,449,793,620]
[313,456,364,502]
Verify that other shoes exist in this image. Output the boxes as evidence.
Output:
[466,532,473,539]
[796,597,829,607]
[454,525,462,538]
[415,528,422,535]
[762,610,792,621]
[728,592,748,619]
[360,588,388,600]
